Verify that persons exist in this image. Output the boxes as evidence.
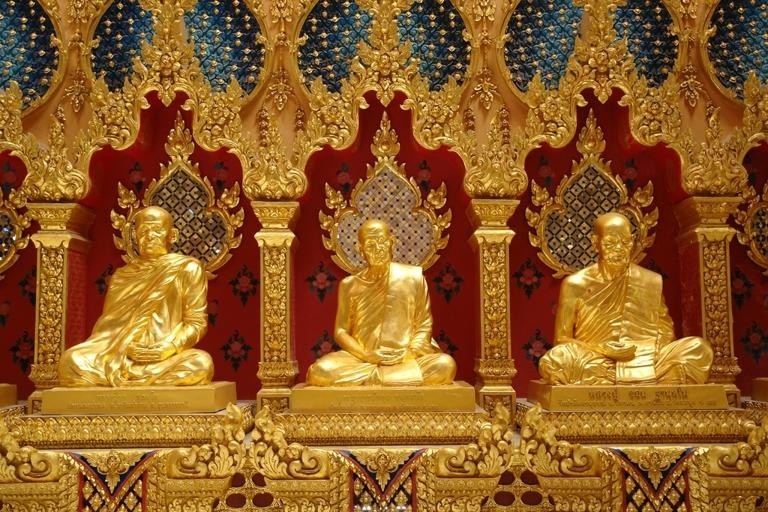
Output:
[57,205,215,390]
[306,219,457,386]
[537,213,715,384]
